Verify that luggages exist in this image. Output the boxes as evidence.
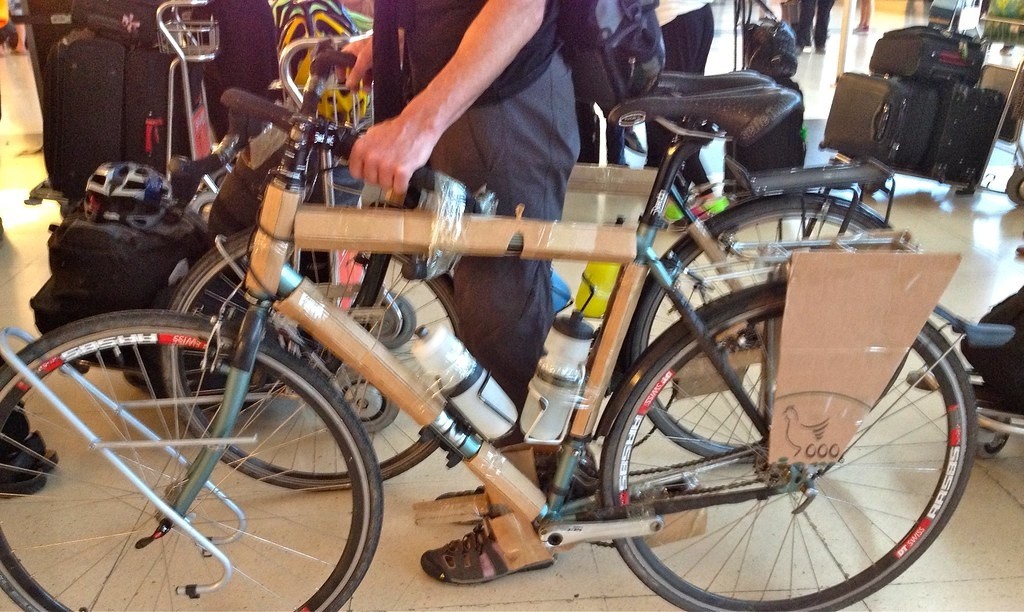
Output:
[869,26,984,87]
[724,0,805,193]
[912,79,1006,186]
[818,71,939,172]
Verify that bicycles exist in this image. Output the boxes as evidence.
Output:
[1,28,1016,609]
[162,0,898,491]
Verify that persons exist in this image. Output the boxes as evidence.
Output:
[337,1,583,586]
[566,1,995,206]
[0,0,21,54]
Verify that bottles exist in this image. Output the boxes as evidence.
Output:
[574,221,628,319]
[519,308,599,445]
[411,318,517,440]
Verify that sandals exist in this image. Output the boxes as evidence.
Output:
[0,450,49,499]
[0,429,59,474]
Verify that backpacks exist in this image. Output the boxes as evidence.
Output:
[549,0,667,116]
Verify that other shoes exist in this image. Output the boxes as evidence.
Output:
[435,486,491,525]
[420,520,559,587]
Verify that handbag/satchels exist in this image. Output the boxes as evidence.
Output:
[738,0,798,84]
